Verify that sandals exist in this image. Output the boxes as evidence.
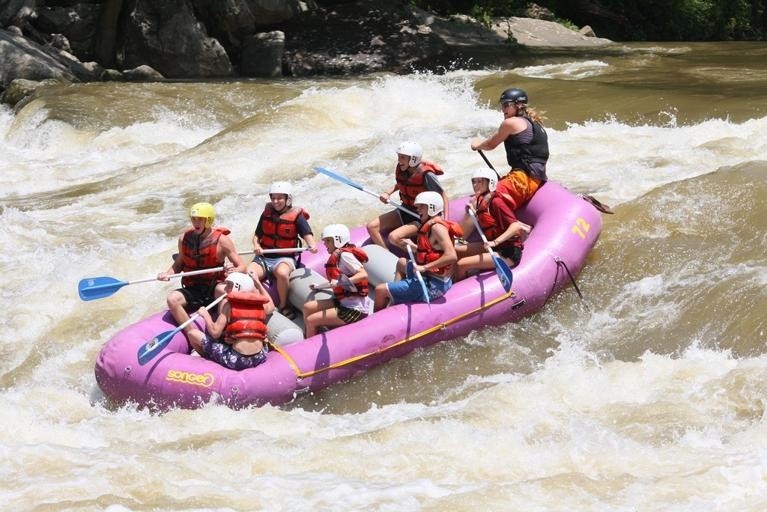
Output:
[277,304,295,321]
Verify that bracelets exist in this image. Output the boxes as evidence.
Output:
[383,191,391,196]
[492,239,498,247]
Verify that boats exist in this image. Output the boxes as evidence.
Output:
[92,177,606,416]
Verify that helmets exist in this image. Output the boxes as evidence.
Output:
[499,88,528,104]
[470,168,499,191]
[320,224,350,249]
[269,181,295,207]
[225,271,255,293]
[395,141,423,167]
[190,203,216,228]
[413,190,444,217]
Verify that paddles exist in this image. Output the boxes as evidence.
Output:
[78,267,223,300]
[314,273,358,293]
[407,245,430,308]
[312,167,422,220]
[137,292,228,366]
[468,208,512,293]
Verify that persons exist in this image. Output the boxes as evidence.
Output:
[469,87,549,208]
[156,201,246,335]
[366,140,450,255]
[450,168,523,284]
[185,268,269,371]
[372,190,459,313]
[247,180,320,321]
[299,221,370,338]
